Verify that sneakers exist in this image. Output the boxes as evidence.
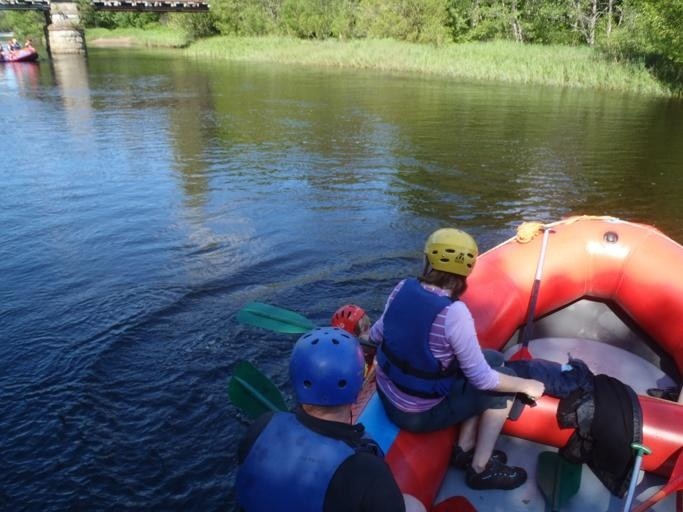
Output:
[452,442,528,490]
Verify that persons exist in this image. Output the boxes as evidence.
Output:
[368,228,544,493]
[8,38,32,54]
[332,304,372,339]
[234,326,406,512]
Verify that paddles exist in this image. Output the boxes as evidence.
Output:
[228,358,290,419]
[631,450,683,511]
[536,451,582,511]
[507,225,556,420]
[235,301,533,400]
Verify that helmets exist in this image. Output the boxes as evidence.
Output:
[423,227,478,277]
[289,304,366,406]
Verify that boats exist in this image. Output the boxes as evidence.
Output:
[330,213,683,511]
[0,45,43,62]
